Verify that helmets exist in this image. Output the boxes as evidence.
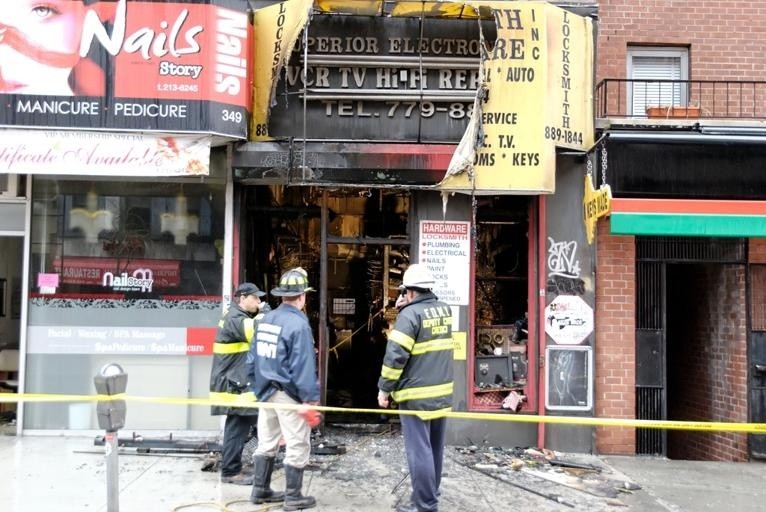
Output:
[403,264,435,289]
[269,269,314,298]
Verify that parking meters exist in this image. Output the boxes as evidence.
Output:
[94,362,127,512]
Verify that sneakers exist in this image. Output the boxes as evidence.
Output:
[222,474,251,485]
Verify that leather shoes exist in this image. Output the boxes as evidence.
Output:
[398,502,437,512]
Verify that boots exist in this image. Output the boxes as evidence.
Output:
[284,463,317,511]
[250,454,285,504]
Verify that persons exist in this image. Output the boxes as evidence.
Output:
[377,263,454,512]
[209,282,272,485]
[246,270,321,511]
[0,0,86,97]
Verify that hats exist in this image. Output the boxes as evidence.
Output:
[233,281,266,298]
